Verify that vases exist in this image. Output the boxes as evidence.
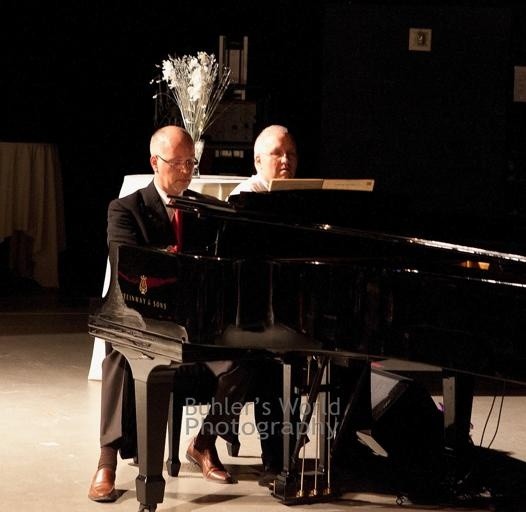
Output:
[192,138,205,178]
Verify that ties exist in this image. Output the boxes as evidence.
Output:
[169,198,182,250]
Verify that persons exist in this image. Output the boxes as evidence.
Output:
[87,125,231,502]
[225,125,309,486]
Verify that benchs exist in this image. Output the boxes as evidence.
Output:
[166,371,332,475]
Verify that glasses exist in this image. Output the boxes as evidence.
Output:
[156,155,198,168]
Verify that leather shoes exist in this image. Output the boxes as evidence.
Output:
[264,465,274,473]
[88,468,116,502]
[186,436,233,484]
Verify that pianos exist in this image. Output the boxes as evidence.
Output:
[87,189,525,512]
